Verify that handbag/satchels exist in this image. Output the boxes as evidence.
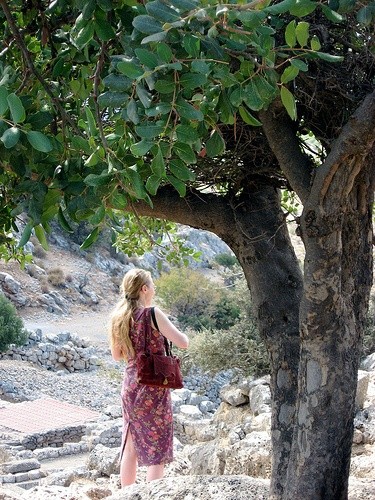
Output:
[138,307,184,390]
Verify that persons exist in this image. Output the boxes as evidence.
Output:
[110,267,191,487]
[24,240,35,265]
